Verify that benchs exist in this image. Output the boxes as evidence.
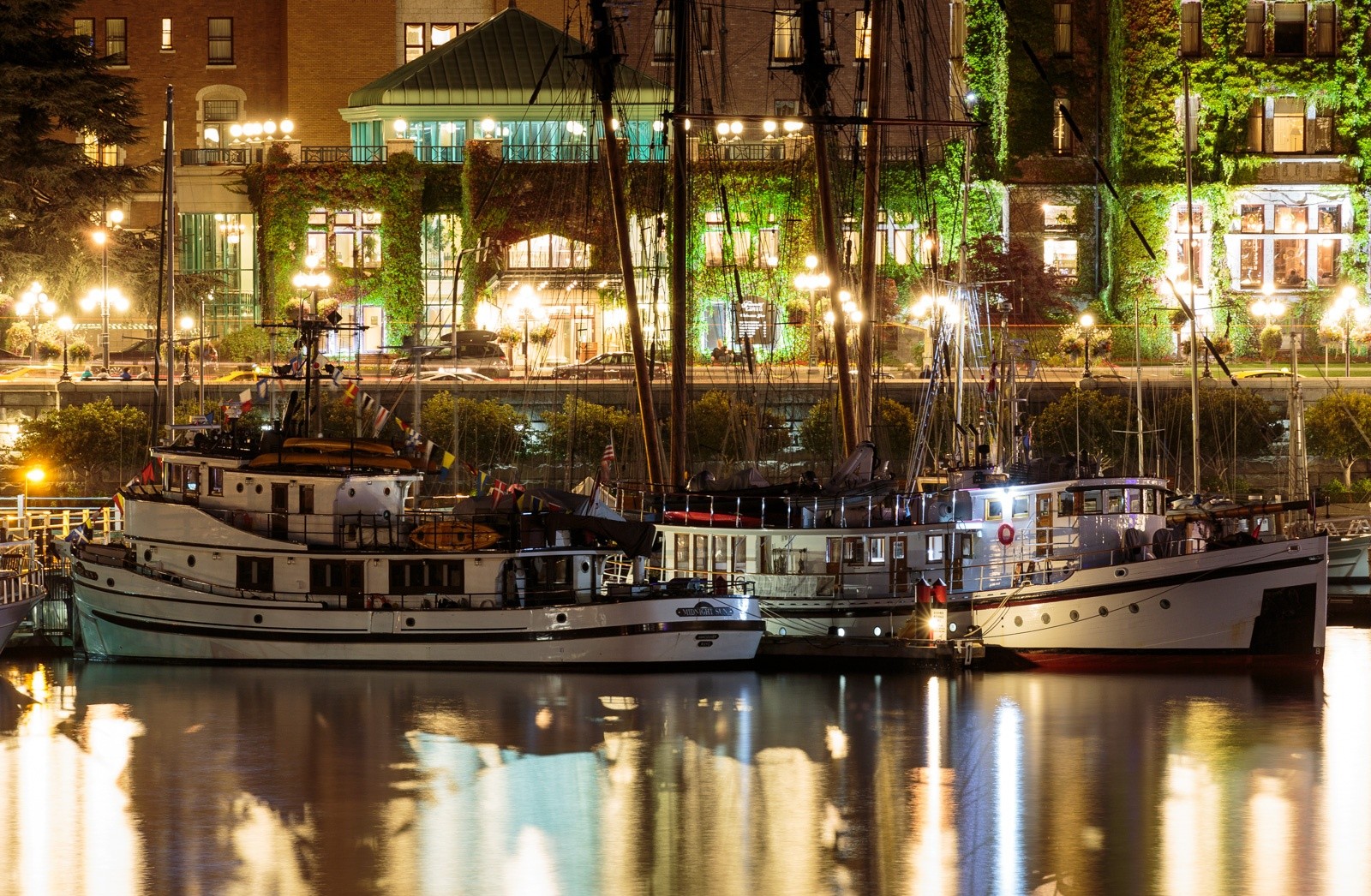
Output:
[829,584,872,598]
[1008,462,1048,485]
[710,351,762,376]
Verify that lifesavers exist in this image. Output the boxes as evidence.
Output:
[998,524,1015,545]
[366,594,387,611]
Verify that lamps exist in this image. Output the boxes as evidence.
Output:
[481,113,495,138]
[393,115,408,139]
[717,120,803,141]
[1289,128,1302,152]
[229,117,295,143]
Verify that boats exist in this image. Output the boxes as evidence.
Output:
[66,82,764,671]
[457,1,1330,664]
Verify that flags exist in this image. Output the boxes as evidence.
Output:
[164,352,617,550]
[65,523,92,547]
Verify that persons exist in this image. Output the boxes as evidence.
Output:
[120,367,133,382]
[718,346,733,364]
[137,365,152,380]
[919,363,932,379]
[799,471,822,497]
[711,339,733,364]
[97,367,110,380]
[81,366,93,382]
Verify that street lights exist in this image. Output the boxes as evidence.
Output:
[16,279,1371,378]
[90,140,126,372]
